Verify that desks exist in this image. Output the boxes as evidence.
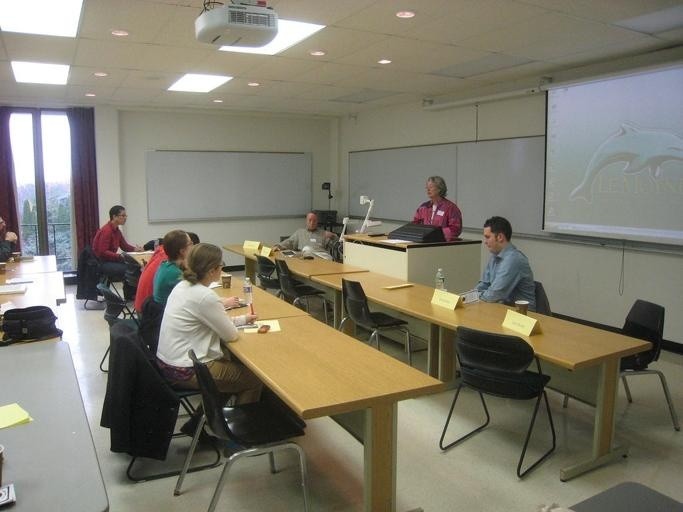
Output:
[130,248,309,322]
[308,270,654,482]
[217,320,448,511]
[0,251,110,511]
[221,236,369,318]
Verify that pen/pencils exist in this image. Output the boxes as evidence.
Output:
[250,304,254,325]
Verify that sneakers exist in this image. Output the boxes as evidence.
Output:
[180,419,211,443]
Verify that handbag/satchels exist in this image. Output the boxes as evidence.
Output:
[0,306,63,347]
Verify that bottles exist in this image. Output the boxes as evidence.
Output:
[154,239,159,252]
[435,268,444,291]
[243,277,253,305]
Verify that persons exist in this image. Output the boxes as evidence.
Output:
[0,217,17,261]
[471,216,536,314]
[412,176,462,239]
[134,230,261,454]
[93,206,141,298]
[270,211,337,255]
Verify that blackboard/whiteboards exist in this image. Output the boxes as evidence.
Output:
[146,148,312,224]
[347,135,683,257]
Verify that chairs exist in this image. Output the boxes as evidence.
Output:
[561,297,681,431]
[252,253,305,297]
[275,261,328,320]
[338,280,413,367]
[78,248,124,313]
[108,319,224,482]
[436,326,556,478]
[533,281,552,316]
[172,348,314,511]
[95,283,144,372]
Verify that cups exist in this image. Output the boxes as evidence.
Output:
[0,262,6,275]
[515,300,529,316]
[0,444,5,487]
[12,252,20,262]
[220,274,232,289]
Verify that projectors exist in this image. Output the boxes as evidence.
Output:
[195,4,278,47]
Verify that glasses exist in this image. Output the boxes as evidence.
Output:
[219,261,224,267]
